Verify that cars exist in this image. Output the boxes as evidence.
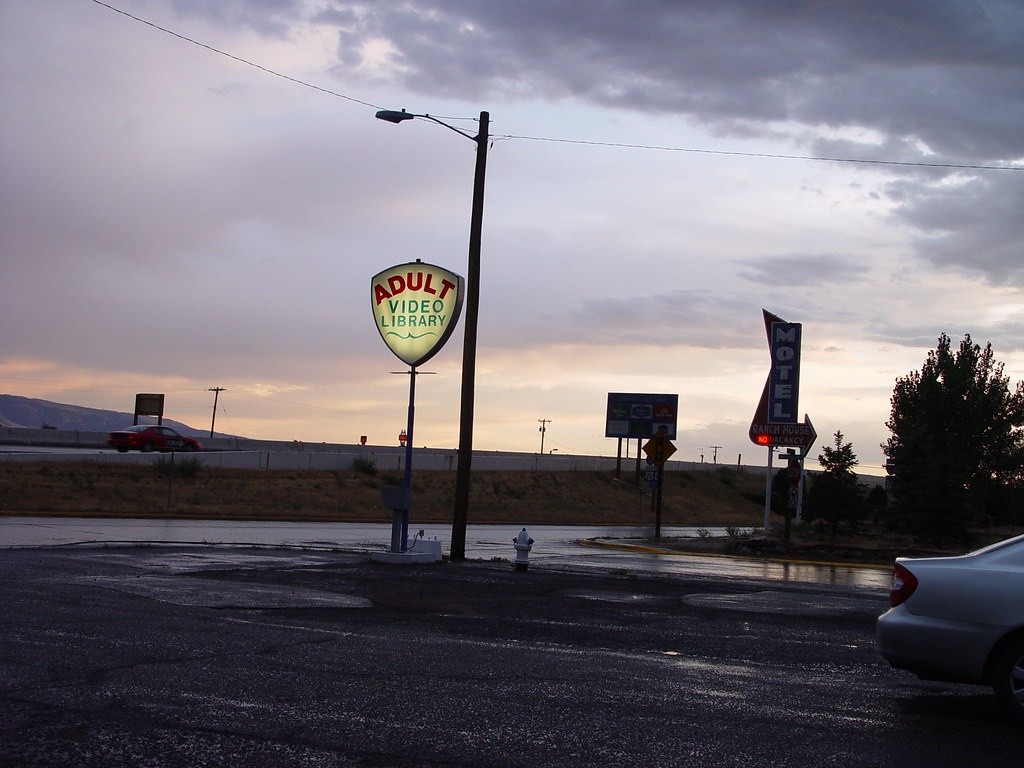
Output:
[875,534,1024,721]
[108,424,204,453]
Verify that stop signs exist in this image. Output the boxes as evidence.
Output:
[789,460,801,486]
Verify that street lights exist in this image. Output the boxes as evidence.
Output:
[374,109,490,560]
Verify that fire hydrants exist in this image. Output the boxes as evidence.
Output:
[512,526,536,571]
